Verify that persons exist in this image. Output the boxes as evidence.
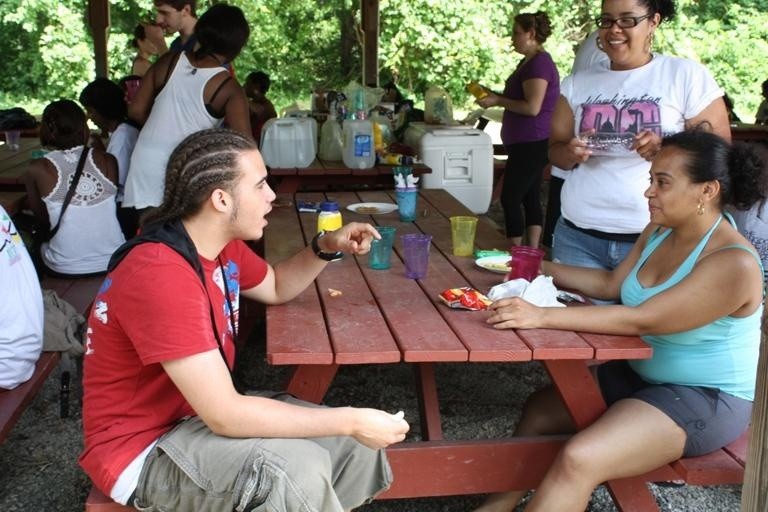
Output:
[474,10,559,248]
[476,119,768,511]
[0,204,45,394]
[242,71,277,137]
[0,73,161,404]
[78,127,411,512]
[547,0,733,313]
[123,0,252,235]
[721,80,768,125]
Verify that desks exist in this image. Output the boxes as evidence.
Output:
[257,158,432,191]
[0,137,42,184]
[262,190,655,512]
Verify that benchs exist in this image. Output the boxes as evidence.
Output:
[661,414,758,510]
[1,272,110,451]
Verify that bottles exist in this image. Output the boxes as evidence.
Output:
[313,86,413,172]
[424,83,450,125]
[575,132,640,155]
[316,201,345,262]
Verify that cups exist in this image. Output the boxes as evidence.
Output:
[5,131,21,151]
[391,167,423,220]
[367,228,397,269]
[509,246,544,285]
[449,215,479,257]
[126,80,142,103]
[401,232,432,278]
[466,81,488,102]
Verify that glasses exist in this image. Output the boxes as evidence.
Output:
[594,12,649,29]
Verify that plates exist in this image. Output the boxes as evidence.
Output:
[474,255,516,274]
[346,202,400,217]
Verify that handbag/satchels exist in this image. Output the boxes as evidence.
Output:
[12,209,46,258]
